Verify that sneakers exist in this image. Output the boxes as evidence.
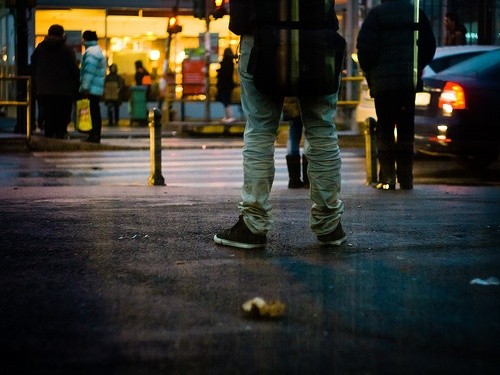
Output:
[213,214,266,250]
[317,219,347,246]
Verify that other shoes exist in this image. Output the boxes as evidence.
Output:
[376,180,395,189]
[400,181,413,189]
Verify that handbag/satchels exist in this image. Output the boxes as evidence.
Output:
[75,98,93,131]
[248,31,347,96]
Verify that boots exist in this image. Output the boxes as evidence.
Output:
[286,153,303,188]
[302,154,310,186]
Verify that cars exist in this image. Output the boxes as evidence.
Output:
[354,43,500,156]
[411,50,500,182]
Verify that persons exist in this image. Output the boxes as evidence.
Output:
[356,0,437,190]
[444,12,467,46]
[214,47,235,107]
[281,96,310,189]
[102,64,124,127]
[135,60,151,90]
[30,24,81,140]
[78,31,107,143]
[213,0,350,250]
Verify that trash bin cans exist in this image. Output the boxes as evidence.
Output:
[130,85,157,126]
